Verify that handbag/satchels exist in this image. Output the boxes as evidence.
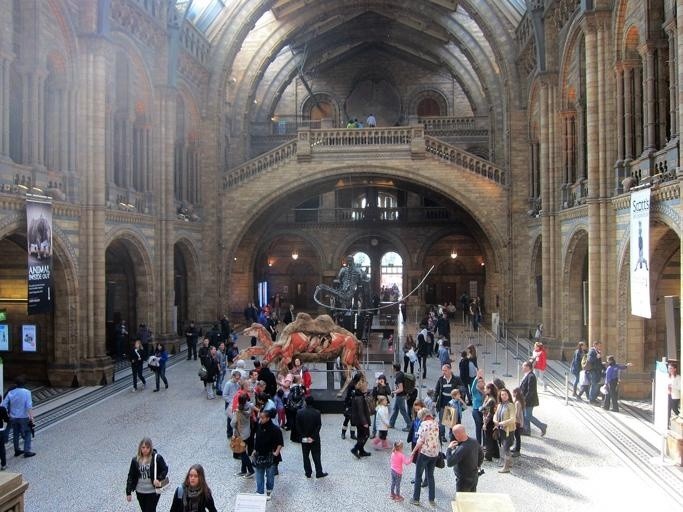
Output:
[231,436,246,453]
[155,477,170,494]
[252,452,273,467]
[436,452,446,468]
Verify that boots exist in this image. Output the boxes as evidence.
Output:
[341,426,357,439]
[373,437,391,448]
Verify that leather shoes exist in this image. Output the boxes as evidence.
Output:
[234,472,255,478]
[316,473,328,477]
[14,450,36,457]
[351,449,370,458]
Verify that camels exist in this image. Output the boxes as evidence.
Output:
[231,311,369,399]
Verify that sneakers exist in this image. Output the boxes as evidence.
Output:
[499,448,520,473]
[267,492,271,501]
[410,498,419,505]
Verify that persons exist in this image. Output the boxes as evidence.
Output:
[388,335,478,443]
[0,394,10,470]
[470,296,482,332]
[1,376,36,458]
[668,362,680,429]
[528,342,546,391]
[470,370,526,473]
[570,342,586,396]
[586,341,602,404]
[409,408,439,506]
[381,283,407,323]
[535,320,543,341]
[445,424,484,492]
[428,292,456,370]
[407,400,428,487]
[519,361,547,436]
[577,356,589,400]
[130,340,146,393]
[170,464,218,512]
[185,320,198,360]
[342,373,393,458]
[198,291,328,500]
[153,343,168,392]
[390,441,413,501]
[601,356,632,412]
[126,437,168,512]
